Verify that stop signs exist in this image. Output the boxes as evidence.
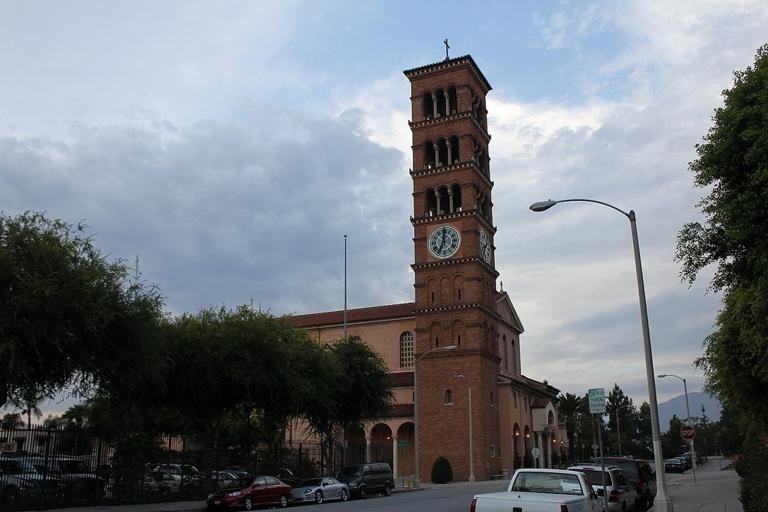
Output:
[679,424,696,440]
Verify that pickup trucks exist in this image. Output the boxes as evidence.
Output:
[469,464,609,512]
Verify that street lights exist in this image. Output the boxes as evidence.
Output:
[411,344,458,488]
[615,402,634,457]
[457,373,478,483]
[529,194,676,512]
[656,372,702,485]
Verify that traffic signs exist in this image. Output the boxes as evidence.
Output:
[587,387,606,414]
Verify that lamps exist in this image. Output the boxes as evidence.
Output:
[512,430,564,445]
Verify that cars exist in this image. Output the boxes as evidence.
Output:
[661,447,704,476]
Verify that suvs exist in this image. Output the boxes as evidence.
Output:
[559,459,640,512]
[592,455,657,512]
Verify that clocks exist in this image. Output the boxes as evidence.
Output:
[479,231,489,263]
[427,223,462,260]
